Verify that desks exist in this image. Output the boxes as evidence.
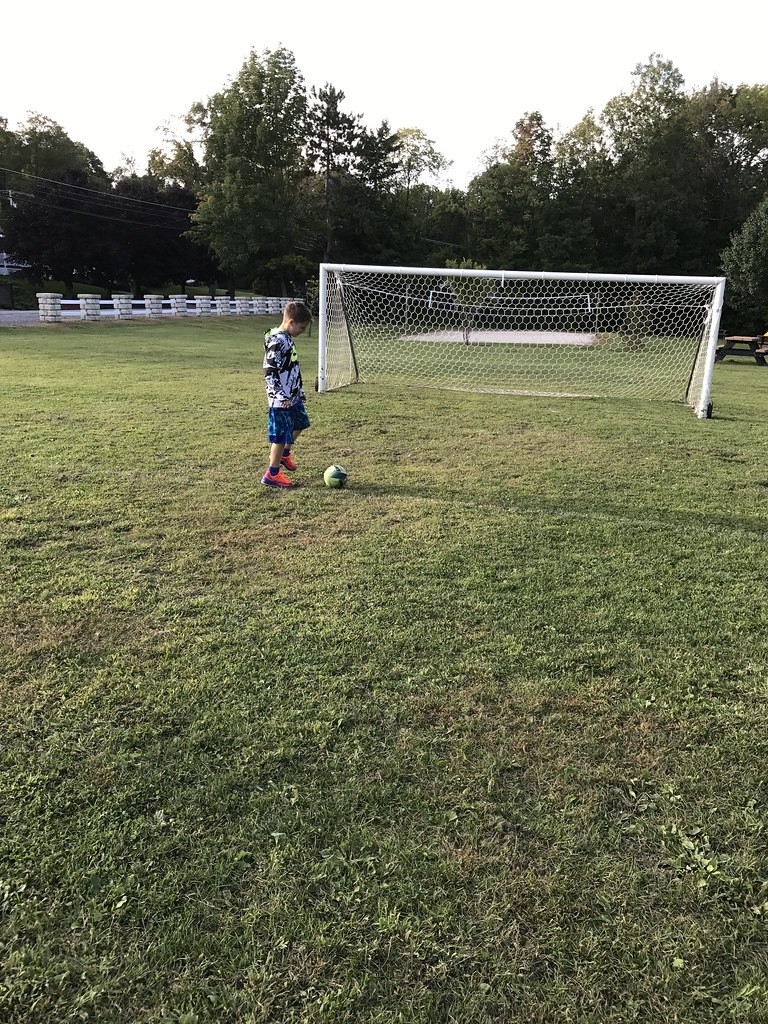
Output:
[714,336,768,366]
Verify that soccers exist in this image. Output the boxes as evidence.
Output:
[324,465,348,490]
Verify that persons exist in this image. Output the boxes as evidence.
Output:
[261,302,311,488]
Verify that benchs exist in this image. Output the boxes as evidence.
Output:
[715,344,724,354]
[754,349,768,357]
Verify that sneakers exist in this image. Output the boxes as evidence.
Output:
[280,451,296,471]
[261,470,292,488]
